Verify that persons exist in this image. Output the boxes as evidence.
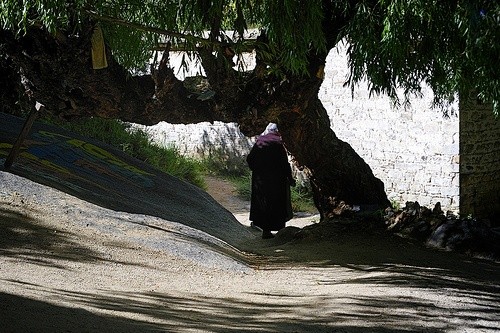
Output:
[245,121,296,239]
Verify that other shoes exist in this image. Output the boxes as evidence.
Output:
[262,233,275,239]
